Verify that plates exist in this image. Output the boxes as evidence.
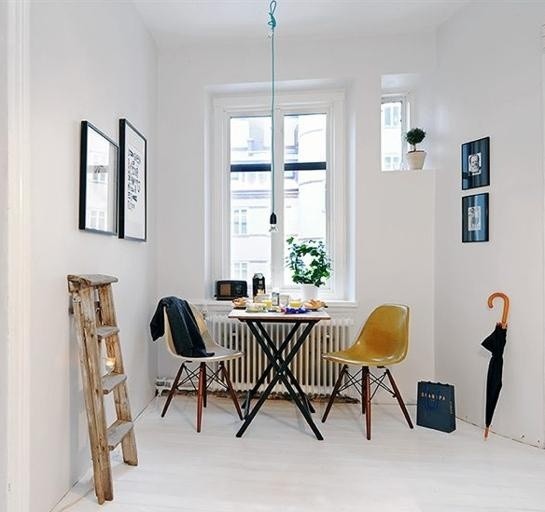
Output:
[234,306,246,309]
[246,309,260,312]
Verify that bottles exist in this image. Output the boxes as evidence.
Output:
[252,273,265,303]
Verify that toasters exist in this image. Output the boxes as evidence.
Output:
[215,280,247,301]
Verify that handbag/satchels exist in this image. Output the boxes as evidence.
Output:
[417,381,456,433]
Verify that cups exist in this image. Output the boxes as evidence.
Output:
[257,292,303,312]
[247,303,259,311]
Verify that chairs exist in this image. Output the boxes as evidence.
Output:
[321,303,414,441]
[160,302,245,434]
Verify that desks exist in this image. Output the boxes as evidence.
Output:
[226,305,332,441]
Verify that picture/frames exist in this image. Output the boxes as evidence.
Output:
[78,116,148,242]
[460,136,493,243]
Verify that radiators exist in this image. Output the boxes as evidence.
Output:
[208,312,353,399]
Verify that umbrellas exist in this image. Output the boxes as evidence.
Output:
[481,293,509,439]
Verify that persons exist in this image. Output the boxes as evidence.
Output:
[469,154,481,176]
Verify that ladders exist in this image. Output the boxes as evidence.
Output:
[67,273,139,506]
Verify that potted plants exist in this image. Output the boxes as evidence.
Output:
[406,127,428,170]
[281,234,334,302]
